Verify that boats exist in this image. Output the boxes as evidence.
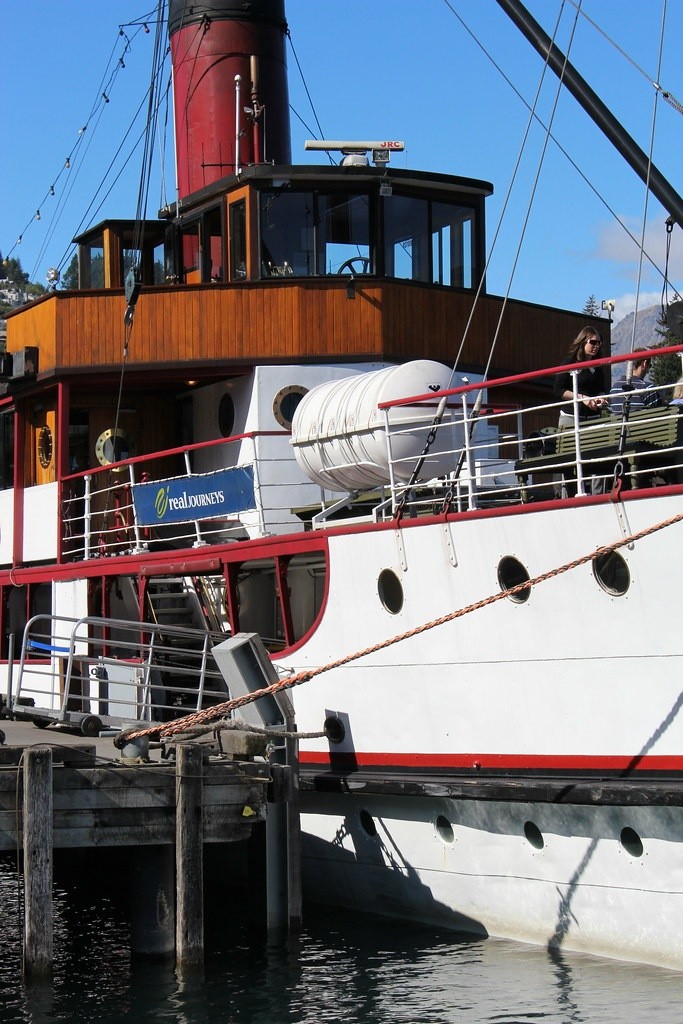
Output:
[0,0,683,978]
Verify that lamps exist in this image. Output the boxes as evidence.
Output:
[380,177,392,196]
[371,148,390,167]
[46,267,60,290]
[601,299,615,319]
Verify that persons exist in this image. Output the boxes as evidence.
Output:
[607,347,666,415]
[668,376,683,406]
[556,324,611,499]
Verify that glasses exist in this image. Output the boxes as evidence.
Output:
[647,361,652,368]
[587,339,603,347]
